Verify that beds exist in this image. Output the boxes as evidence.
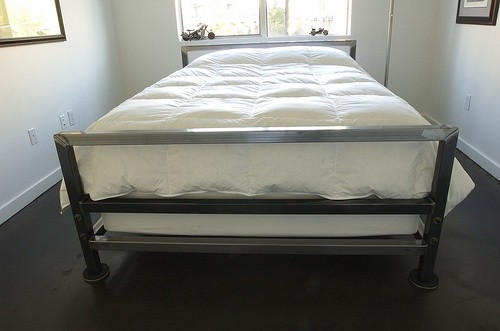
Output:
[49,44,475,293]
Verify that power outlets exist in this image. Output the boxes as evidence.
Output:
[29,129,38,146]
[68,110,75,126]
[464,95,472,111]
[59,113,68,131]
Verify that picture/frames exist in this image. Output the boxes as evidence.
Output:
[0,0,67,48]
[455,0,500,27]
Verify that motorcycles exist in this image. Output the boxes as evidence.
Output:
[309,27,328,36]
[180,23,215,41]
